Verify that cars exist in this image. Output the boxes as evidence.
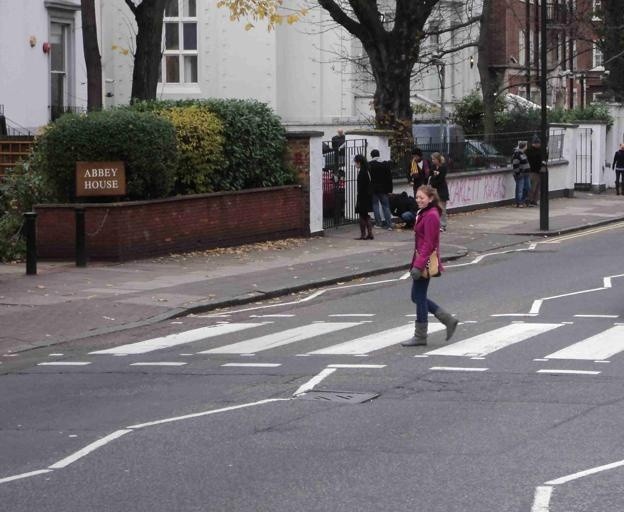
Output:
[322,123,507,217]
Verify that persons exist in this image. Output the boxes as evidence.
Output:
[332,127,345,151]
[407,148,430,199]
[400,184,459,348]
[512,142,535,208]
[428,152,449,233]
[353,153,377,241]
[387,191,420,229]
[369,151,394,229]
[524,135,542,205]
[611,145,624,196]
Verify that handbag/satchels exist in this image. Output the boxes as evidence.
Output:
[415,251,438,278]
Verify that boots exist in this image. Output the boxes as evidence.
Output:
[434,307,459,340]
[401,320,428,346]
[360,224,373,240]
